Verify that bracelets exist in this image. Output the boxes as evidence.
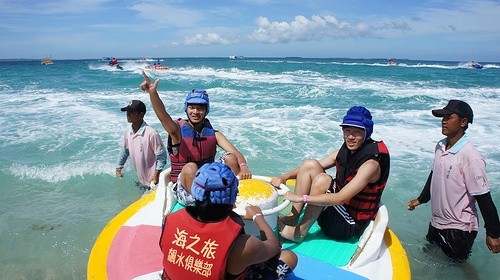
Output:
[252,213,263,222]
[303,195,308,203]
[239,163,247,167]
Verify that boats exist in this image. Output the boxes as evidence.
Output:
[387,62,398,66]
[468,64,484,70]
[142,63,170,71]
[41,55,54,65]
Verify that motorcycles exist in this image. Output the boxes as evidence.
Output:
[109,60,123,71]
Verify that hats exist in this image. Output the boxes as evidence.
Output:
[121,100,146,114]
[184,89,209,115]
[431,100,474,124]
[191,162,238,205]
[340,106,374,143]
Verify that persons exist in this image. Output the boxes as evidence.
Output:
[115,100,167,192]
[271,106,390,243]
[159,162,298,280]
[138,71,251,207]
[406,100,500,263]
[110,57,162,69]
